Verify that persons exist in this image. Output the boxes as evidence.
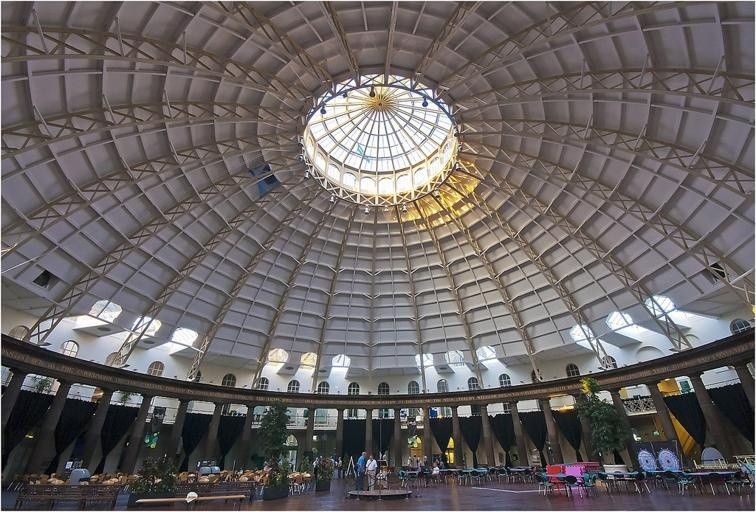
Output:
[264,451,445,492]
[72,457,82,469]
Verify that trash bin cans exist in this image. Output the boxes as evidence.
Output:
[70,469,91,486]
[198,466,220,481]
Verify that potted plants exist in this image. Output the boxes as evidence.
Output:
[310,454,335,492]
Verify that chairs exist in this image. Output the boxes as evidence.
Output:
[222,410,292,429]
[393,460,755,501]
[6,466,316,511]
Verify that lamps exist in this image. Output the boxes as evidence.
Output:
[295,72,464,215]
